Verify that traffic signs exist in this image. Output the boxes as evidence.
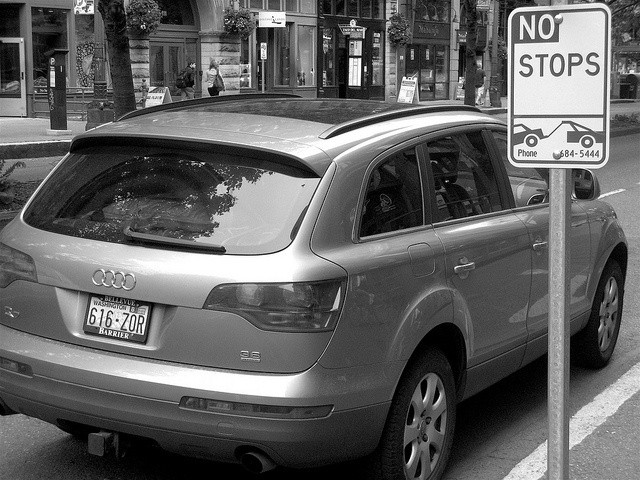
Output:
[260,42,268,94]
[506,3,612,480]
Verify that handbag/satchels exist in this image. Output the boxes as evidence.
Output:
[213,69,224,92]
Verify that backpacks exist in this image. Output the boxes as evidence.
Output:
[176,68,192,88]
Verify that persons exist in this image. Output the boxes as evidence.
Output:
[180,61,196,101]
[475,64,487,105]
[205,59,226,96]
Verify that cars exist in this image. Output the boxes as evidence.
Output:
[512,125,544,147]
[561,121,603,148]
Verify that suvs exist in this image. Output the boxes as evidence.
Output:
[0,93,629,480]
